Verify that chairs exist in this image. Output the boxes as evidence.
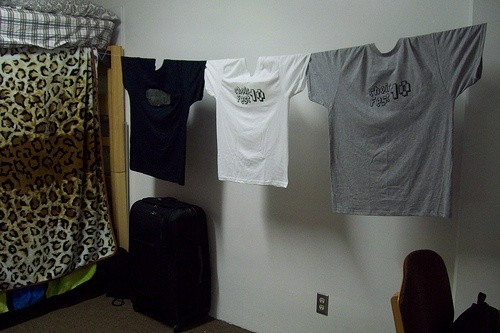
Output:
[390,250,454,333]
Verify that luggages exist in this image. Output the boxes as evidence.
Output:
[130,196,211,332]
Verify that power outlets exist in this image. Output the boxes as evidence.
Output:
[316,293,328,316]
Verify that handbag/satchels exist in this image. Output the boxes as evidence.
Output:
[107,270,134,306]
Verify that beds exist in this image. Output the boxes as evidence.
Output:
[0,46,130,313]
